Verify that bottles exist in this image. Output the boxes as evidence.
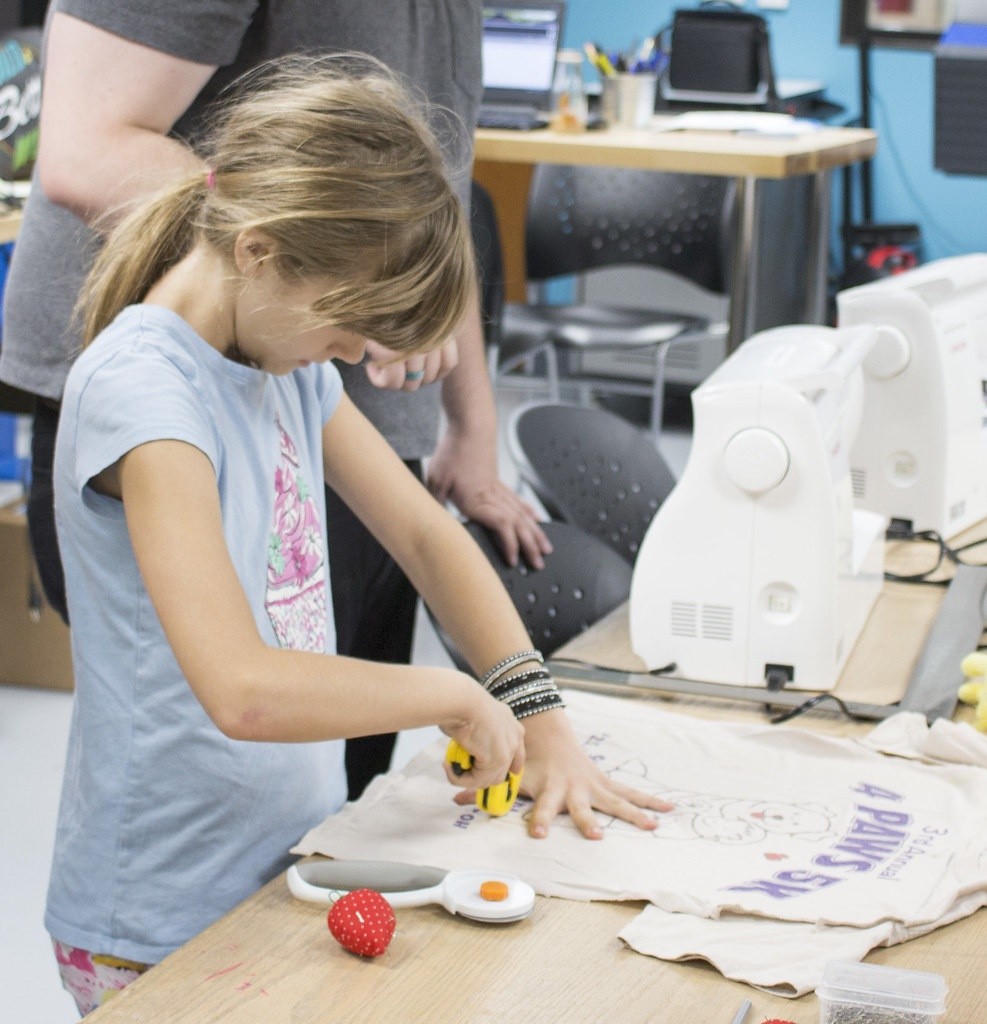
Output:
[547,45,590,136]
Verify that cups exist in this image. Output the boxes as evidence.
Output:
[601,68,655,128]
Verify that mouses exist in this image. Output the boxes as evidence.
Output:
[585,114,604,130]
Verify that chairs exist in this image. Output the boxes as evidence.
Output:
[423,517,633,686]
[506,397,677,568]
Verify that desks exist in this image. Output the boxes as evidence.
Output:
[74,521,987,1024]
[473,116,877,359]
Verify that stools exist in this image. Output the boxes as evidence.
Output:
[489,297,709,443]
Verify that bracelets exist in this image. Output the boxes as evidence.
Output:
[481,650,566,720]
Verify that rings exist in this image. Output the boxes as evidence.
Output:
[406,368,426,381]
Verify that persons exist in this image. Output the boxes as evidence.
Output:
[0,0,554,802]
[44,47,679,1024]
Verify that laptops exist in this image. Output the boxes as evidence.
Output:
[474,20,562,130]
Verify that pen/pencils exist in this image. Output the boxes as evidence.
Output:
[584,35,671,77]
